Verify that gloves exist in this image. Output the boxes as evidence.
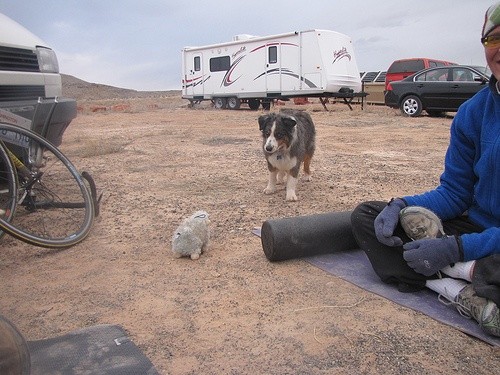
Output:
[374,198,406,247]
[402,236,462,276]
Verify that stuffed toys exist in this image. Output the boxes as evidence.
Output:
[172,211,211,260]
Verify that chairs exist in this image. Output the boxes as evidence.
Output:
[460,72,474,81]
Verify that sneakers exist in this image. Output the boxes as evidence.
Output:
[438,283,500,337]
[399,207,446,241]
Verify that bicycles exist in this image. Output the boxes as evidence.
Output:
[0,121,99,248]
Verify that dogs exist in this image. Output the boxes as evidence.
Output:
[256,108,317,203]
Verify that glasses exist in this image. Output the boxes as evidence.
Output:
[481,32,500,47]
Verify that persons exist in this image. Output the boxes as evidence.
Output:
[351,0,500,339]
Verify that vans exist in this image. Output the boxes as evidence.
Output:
[383,57,473,95]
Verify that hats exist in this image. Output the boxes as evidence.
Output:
[478,0,500,41]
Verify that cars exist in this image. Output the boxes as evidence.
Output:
[384,64,493,117]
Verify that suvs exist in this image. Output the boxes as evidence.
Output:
[0,11,78,183]
